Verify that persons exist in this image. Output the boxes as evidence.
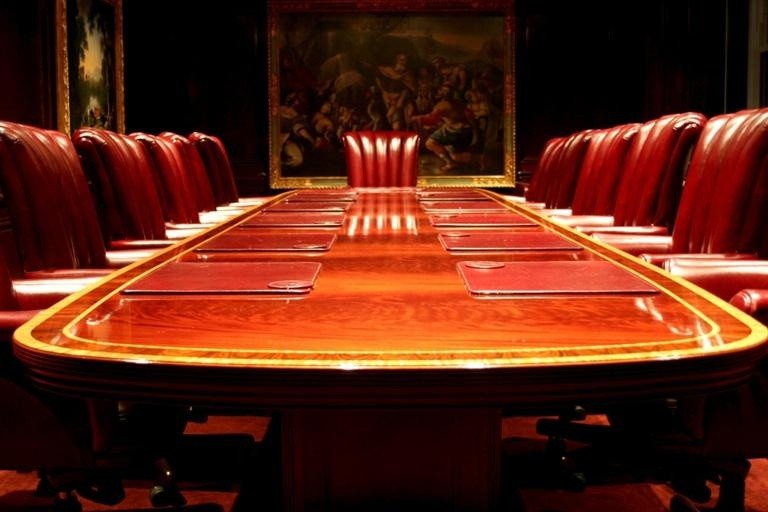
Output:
[283,43,504,172]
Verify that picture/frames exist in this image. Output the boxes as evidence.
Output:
[54,2,125,135]
[265,2,516,189]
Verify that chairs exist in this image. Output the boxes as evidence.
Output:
[340,126,424,189]
[1,121,239,510]
[525,105,767,512]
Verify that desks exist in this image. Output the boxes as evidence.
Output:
[12,153,766,510]
